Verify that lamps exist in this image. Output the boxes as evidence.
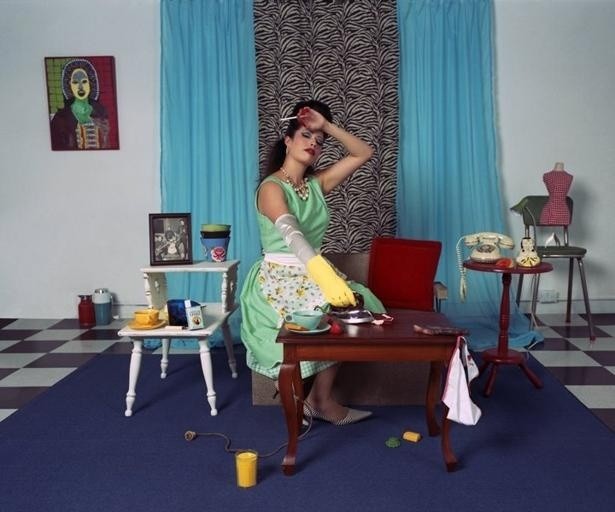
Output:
[509,196,538,253]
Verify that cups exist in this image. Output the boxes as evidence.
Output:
[199,225,231,264]
[235,446,259,487]
[286,310,322,330]
[133,309,159,324]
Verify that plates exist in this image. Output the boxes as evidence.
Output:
[285,320,332,335]
[128,318,166,330]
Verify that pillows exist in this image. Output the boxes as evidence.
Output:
[367,236,448,312]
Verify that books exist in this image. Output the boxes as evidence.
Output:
[413,318,471,338]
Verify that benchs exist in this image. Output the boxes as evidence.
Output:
[250,252,444,408]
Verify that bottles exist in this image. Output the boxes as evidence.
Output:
[76,288,111,328]
[515,237,541,268]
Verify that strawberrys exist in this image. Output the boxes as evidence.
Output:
[329,321,342,334]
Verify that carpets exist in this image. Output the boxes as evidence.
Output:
[1,351,613,512]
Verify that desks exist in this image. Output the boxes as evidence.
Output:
[117,260,242,419]
[462,258,553,397]
[275,311,466,476]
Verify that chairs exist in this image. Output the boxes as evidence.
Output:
[520,195,598,342]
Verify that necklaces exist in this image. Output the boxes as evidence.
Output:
[280,167,310,201]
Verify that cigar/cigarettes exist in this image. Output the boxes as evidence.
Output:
[278,114,307,122]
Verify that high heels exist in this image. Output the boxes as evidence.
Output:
[272,380,310,433]
[303,396,374,427]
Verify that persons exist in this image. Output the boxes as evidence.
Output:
[165,231,186,259]
[538,160,574,225]
[237,99,380,426]
[154,235,164,260]
[49,59,112,149]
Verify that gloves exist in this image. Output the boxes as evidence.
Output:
[305,254,358,310]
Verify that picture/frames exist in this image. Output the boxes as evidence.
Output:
[147,211,193,264]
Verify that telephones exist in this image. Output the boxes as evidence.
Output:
[463,232,515,263]
